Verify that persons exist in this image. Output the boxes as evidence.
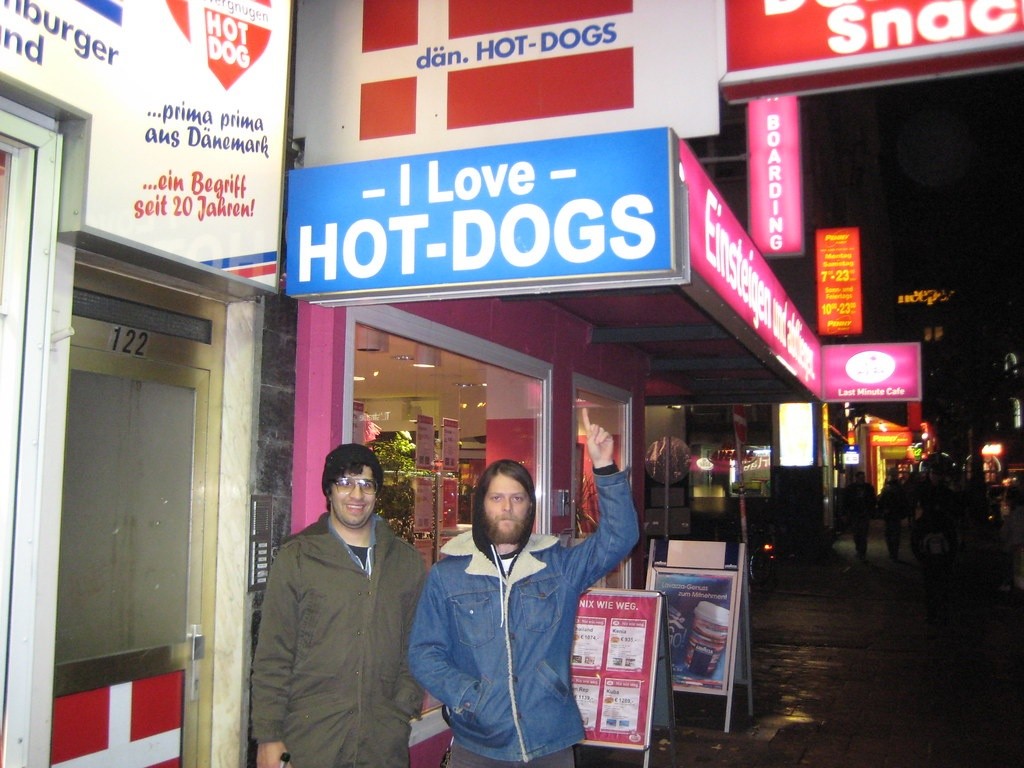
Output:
[877,470,910,558]
[408,408,639,768]
[913,456,1024,622]
[250,444,427,768]
[845,471,877,559]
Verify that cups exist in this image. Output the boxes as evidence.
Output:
[684,599,730,676]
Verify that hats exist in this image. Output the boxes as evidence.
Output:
[322,443,383,498]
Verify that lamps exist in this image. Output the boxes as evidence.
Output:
[412,344,439,367]
[356,326,380,351]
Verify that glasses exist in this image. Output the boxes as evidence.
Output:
[332,477,379,495]
